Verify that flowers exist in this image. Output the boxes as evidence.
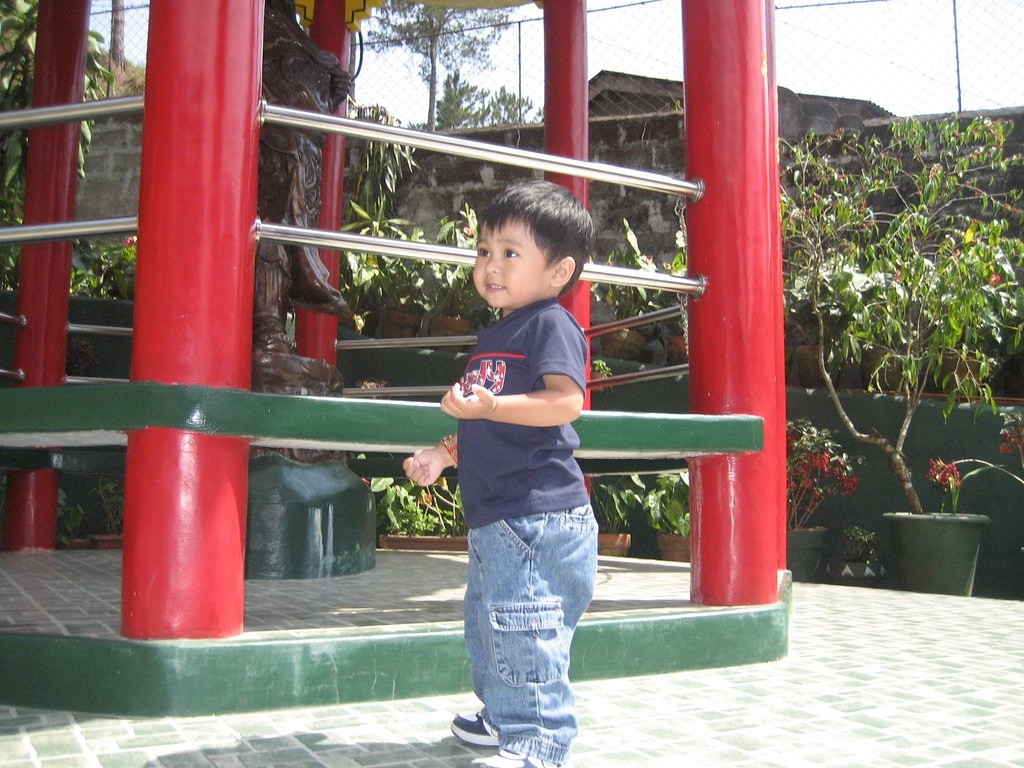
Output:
[786,417,865,525]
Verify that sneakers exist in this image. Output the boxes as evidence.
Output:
[472,749,557,768]
[451,711,499,745]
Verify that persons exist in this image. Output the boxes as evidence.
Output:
[402,180,599,767]
[251,0,351,351]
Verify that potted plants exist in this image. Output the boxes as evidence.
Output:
[370,476,468,551]
[591,472,646,556]
[787,525,828,583]
[642,472,691,563]
[785,115,1023,598]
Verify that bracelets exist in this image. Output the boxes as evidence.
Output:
[442,436,457,464]
